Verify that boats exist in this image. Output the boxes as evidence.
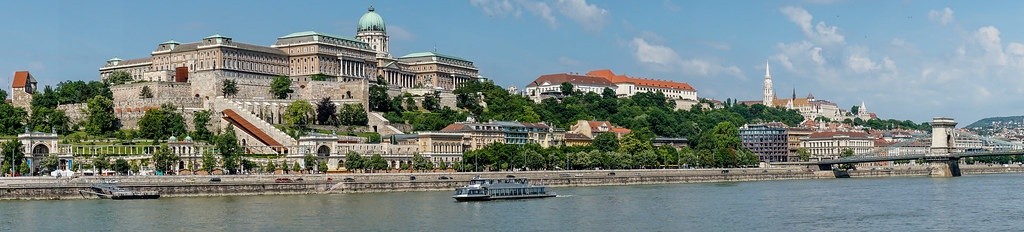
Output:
[90,184,162,201]
[452,175,557,202]
